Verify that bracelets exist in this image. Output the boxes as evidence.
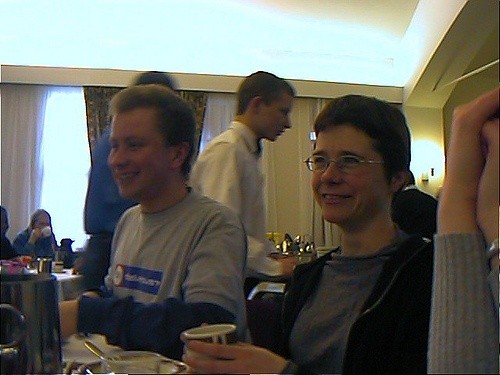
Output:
[485,236,499,273]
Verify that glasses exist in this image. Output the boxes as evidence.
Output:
[304,153,387,175]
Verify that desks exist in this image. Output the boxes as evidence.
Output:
[29,268,86,301]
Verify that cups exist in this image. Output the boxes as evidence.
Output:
[104,351,161,373]
[40,226,51,236]
[185,325,238,344]
[71,333,106,349]
[54,262,63,272]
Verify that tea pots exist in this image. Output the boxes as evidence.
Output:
[281,233,294,255]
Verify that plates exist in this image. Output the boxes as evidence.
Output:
[62,343,122,363]
[79,357,189,375]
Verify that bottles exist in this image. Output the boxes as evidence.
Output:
[38,258,49,273]
[306,242,315,254]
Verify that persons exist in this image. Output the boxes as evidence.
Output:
[428,85,499,375]
[12,209,60,262]
[59,84,254,361]
[188,71,303,302]
[0,206,21,261]
[392,170,438,242]
[79,71,178,298]
[183,94,434,374]
